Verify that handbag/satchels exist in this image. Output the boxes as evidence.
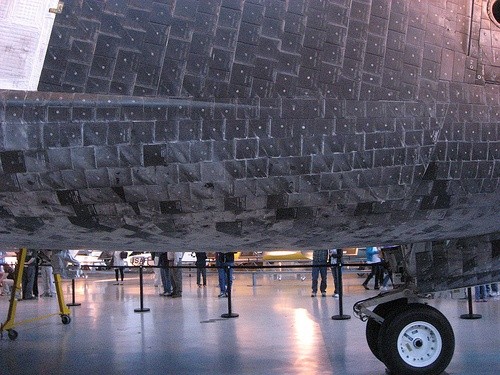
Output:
[120,251,127,259]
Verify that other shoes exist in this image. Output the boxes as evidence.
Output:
[322,292,326,297]
[197,284,202,287]
[35,294,38,297]
[475,298,479,302]
[218,293,228,297]
[159,291,169,296]
[170,292,182,298]
[113,282,119,285]
[120,281,124,285]
[332,293,339,298]
[203,284,207,287]
[374,286,380,290]
[40,293,52,297]
[379,286,388,291]
[311,292,316,297]
[23,296,35,300]
[154,283,158,287]
[482,298,487,302]
[362,284,369,290]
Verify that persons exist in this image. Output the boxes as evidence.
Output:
[154,251,163,287]
[114,251,126,286]
[159,252,174,296]
[362,247,500,302]
[0,249,57,299]
[195,252,209,287]
[168,252,183,298]
[330,249,341,297]
[216,253,233,297]
[310,250,329,296]
